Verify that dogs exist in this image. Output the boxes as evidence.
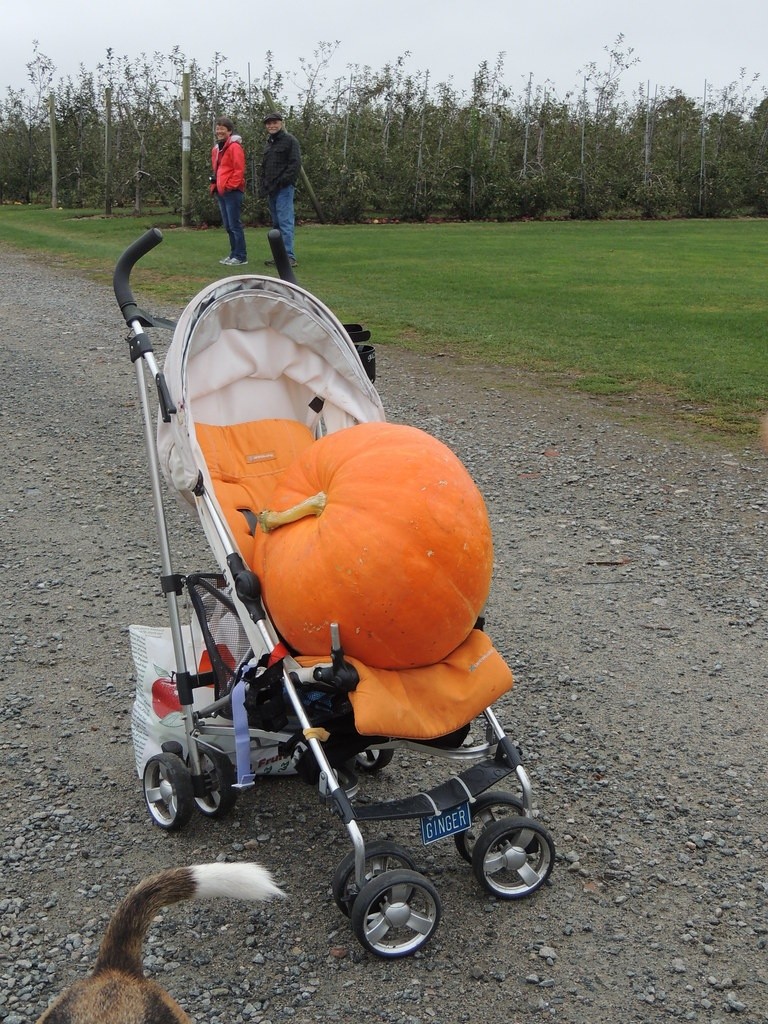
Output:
[33,861,292,1024]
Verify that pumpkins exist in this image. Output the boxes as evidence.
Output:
[251,423,493,670]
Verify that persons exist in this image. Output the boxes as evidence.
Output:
[260,113,300,267]
[207,116,249,266]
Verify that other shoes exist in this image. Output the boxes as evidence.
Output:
[289,258,297,267]
[265,259,276,266]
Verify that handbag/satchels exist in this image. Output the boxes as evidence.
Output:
[128,587,316,780]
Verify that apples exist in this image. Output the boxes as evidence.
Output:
[198,643,236,689]
[152,671,184,719]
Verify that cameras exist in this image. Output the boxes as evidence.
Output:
[209,173,216,183]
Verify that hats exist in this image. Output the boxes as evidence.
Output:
[263,112,282,124]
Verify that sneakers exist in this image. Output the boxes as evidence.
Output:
[220,257,248,266]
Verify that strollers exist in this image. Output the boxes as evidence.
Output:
[108,223,557,961]
